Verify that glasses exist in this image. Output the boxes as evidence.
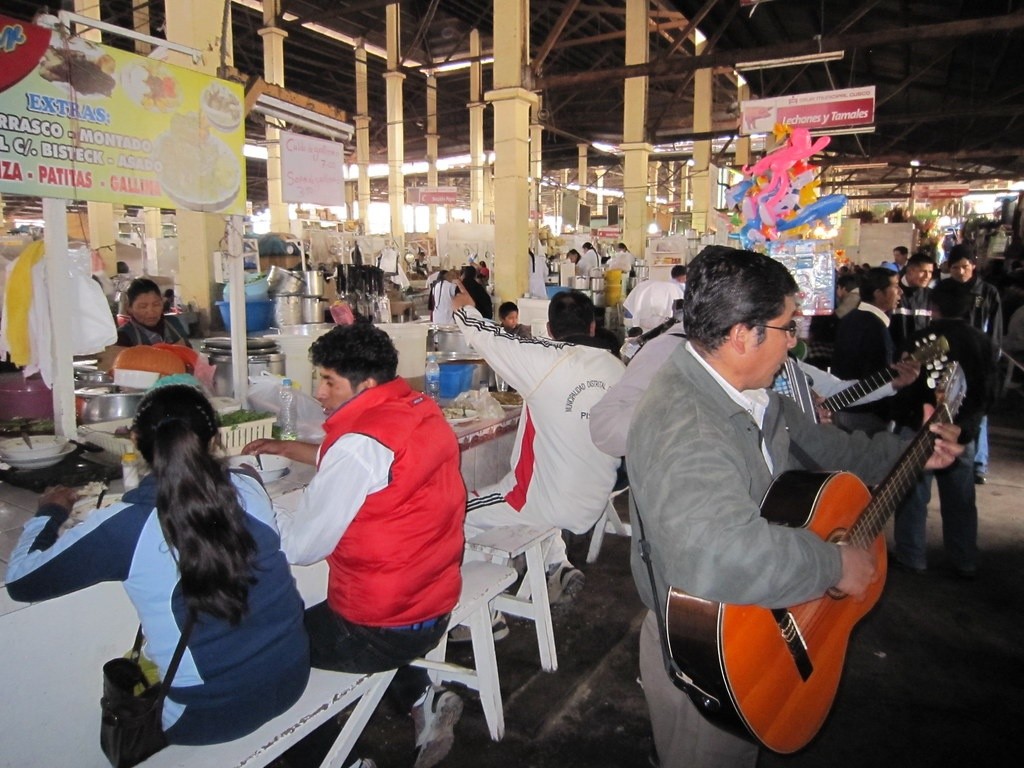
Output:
[719,317,797,338]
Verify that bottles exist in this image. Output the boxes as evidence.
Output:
[425,355,441,400]
[120,453,139,492]
[279,379,297,441]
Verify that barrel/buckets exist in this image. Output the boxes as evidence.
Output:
[606,269,621,306]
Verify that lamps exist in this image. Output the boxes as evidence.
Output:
[250,93,356,143]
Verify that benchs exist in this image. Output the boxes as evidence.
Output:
[130,478,635,768]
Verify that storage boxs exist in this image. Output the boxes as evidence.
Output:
[212,300,277,331]
[424,362,478,397]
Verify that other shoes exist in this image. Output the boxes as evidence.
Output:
[447,610,510,641]
[974,475,984,484]
[522,562,585,632]
[945,552,982,577]
[888,552,929,576]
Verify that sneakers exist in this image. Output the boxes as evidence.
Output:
[408,684,463,768]
[349,757,376,768]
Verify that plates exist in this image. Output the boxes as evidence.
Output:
[439,407,477,425]
[491,393,523,410]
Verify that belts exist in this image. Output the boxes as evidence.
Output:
[384,617,438,631]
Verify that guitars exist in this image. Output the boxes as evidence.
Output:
[663,356,974,757]
[819,333,952,414]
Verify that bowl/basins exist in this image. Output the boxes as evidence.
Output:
[229,455,292,483]
[0,436,77,470]
[69,494,128,523]
[214,298,274,332]
[224,272,269,302]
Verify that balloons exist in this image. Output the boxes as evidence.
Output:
[713,121,847,250]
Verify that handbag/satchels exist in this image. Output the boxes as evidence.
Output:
[987,226,1013,260]
[100,657,166,768]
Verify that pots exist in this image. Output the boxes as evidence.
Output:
[627,259,650,292]
[266,265,324,327]
[545,254,605,307]
[207,353,286,399]
[74,387,151,425]
[426,325,481,361]
[75,369,111,382]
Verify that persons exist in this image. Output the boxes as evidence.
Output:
[4,385,311,746]
[111,279,192,350]
[498,302,530,338]
[670,265,687,290]
[807,243,1024,575]
[625,251,965,768]
[590,244,920,458]
[163,289,174,312]
[447,279,627,641]
[229,316,467,768]
[412,252,492,322]
[566,242,635,277]
[294,254,311,271]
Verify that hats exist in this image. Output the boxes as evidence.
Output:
[947,244,977,267]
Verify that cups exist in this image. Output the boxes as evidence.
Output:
[494,371,508,391]
